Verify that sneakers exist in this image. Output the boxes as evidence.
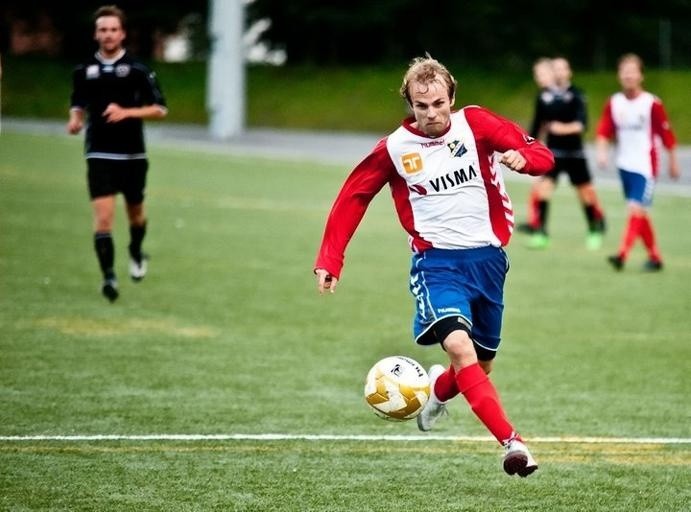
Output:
[502,434,538,479]
[128,252,147,282]
[605,255,664,274]
[416,364,448,432]
[513,218,607,252]
[101,281,120,304]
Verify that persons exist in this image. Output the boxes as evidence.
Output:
[595,52,683,274]
[66,5,170,302]
[312,53,556,479]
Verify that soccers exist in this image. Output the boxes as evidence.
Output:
[364,357,431,421]
